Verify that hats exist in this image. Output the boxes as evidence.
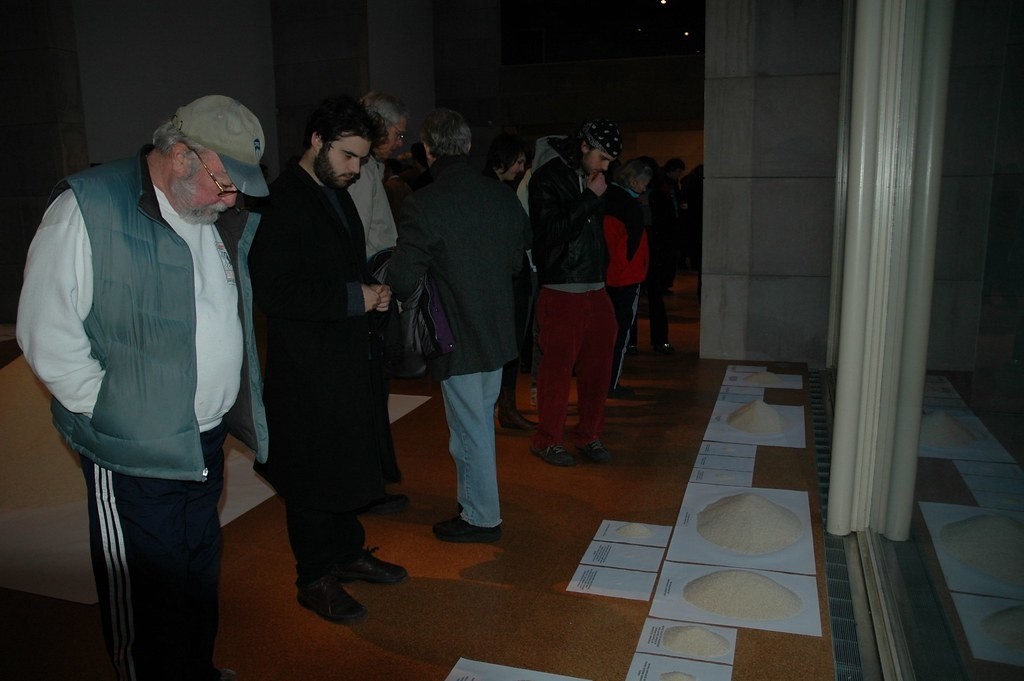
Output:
[171,95,270,197]
[580,117,624,158]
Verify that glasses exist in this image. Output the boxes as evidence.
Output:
[176,141,238,197]
[381,117,409,144]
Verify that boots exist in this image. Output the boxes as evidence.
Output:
[497,388,540,431]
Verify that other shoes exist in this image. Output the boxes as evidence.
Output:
[353,494,410,515]
[432,516,502,543]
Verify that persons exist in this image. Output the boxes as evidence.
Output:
[382,142,428,379]
[386,108,524,543]
[348,91,410,514]
[527,118,618,466]
[483,133,703,430]
[248,96,407,624]
[16,95,270,681]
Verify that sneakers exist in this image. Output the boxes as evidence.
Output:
[529,442,576,466]
[575,439,613,464]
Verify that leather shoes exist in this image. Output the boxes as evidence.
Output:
[331,543,409,583]
[296,574,368,624]
